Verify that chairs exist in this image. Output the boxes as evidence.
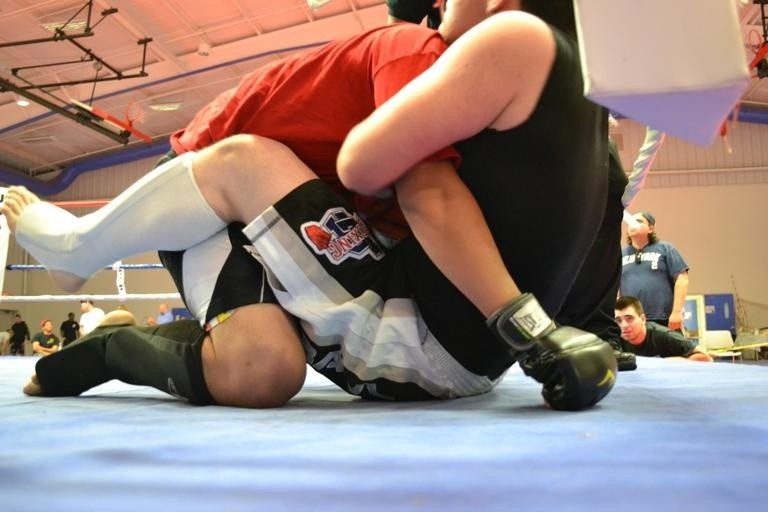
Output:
[703,329,743,364]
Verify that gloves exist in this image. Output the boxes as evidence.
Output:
[485,291,620,413]
